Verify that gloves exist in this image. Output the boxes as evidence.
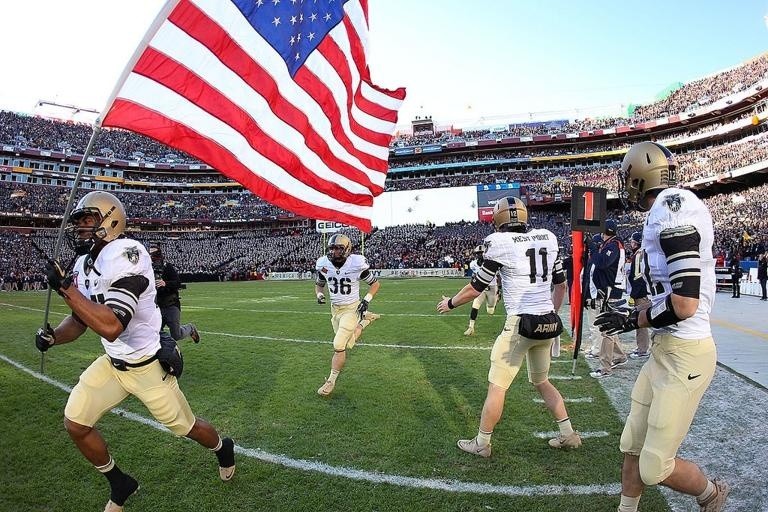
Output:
[42,258,71,297]
[594,307,639,336]
[318,295,326,304]
[357,299,368,311]
[36,323,56,352]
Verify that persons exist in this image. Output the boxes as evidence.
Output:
[436,194,585,461]
[0,50,768,301]
[35,190,237,511]
[147,246,201,344]
[463,219,655,379]
[593,139,730,512]
[316,233,380,396]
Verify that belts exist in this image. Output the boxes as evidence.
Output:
[108,355,157,371]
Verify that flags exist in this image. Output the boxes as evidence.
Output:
[101,0,408,234]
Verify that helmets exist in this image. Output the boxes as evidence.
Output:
[326,233,352,262]
[617,142,679,214]
[62,191,126,255]
[474,245,484,253]
[493,196,527,232]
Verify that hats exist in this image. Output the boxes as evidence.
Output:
[593,219,642,245]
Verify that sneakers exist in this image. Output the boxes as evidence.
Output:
[457,435,492,458]
[190,327,199,343]
[548,430,582,448]
[215,438,235,481]
[104,476,140,512]
[464,327,475,336]
[363,311,380,323]
[696,479,729,512]
[318,377,335,397]
[585,346,649,379]
